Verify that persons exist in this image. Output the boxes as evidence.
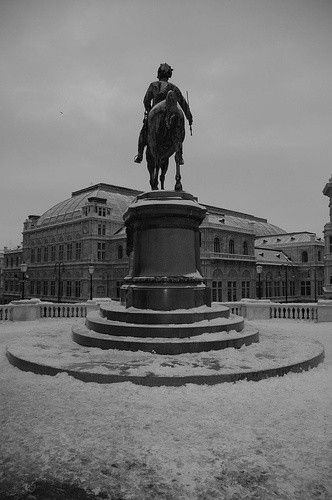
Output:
[134,63,193,163]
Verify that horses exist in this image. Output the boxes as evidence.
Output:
[145,90,184,191]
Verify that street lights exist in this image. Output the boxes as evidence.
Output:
[52,261,65,302]
[255,264,264,299]
[307,269,317,302]
[280,262,294,302]
[88,264,96,299]
[19,260,28,299]
[102,271,109,297]
